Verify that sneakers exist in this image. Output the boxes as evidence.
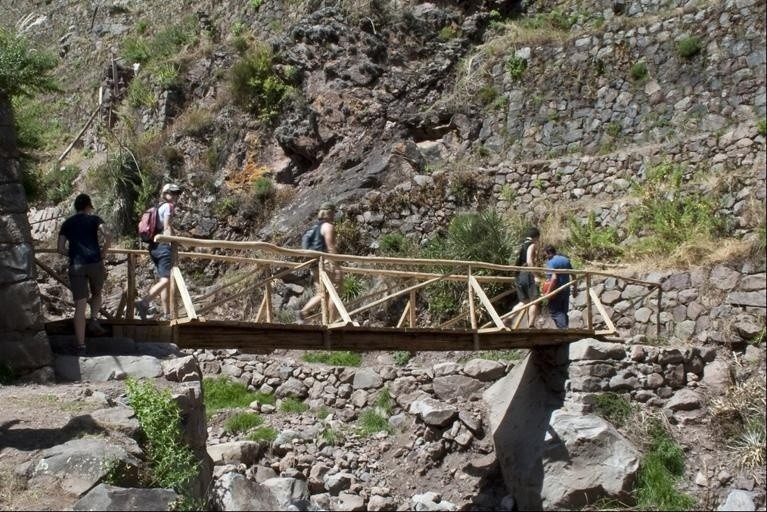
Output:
[133,300,173,322]
[72,319,107,357]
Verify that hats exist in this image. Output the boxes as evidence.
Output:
[318,201,339,213]
[162,183,183,196]
[73,193,96,210]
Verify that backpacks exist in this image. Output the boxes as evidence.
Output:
[508,240,532,274]
[301,222,326,253]
[138,202,170,244]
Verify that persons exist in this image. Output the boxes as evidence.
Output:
[495,226,541,330]
[542,243,579,328]
[295,201,344,326]
[134,182,183,321]
[101,59,140,123]
[55,193,115,353]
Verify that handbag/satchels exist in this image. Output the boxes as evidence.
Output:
[542,278,558,300]
